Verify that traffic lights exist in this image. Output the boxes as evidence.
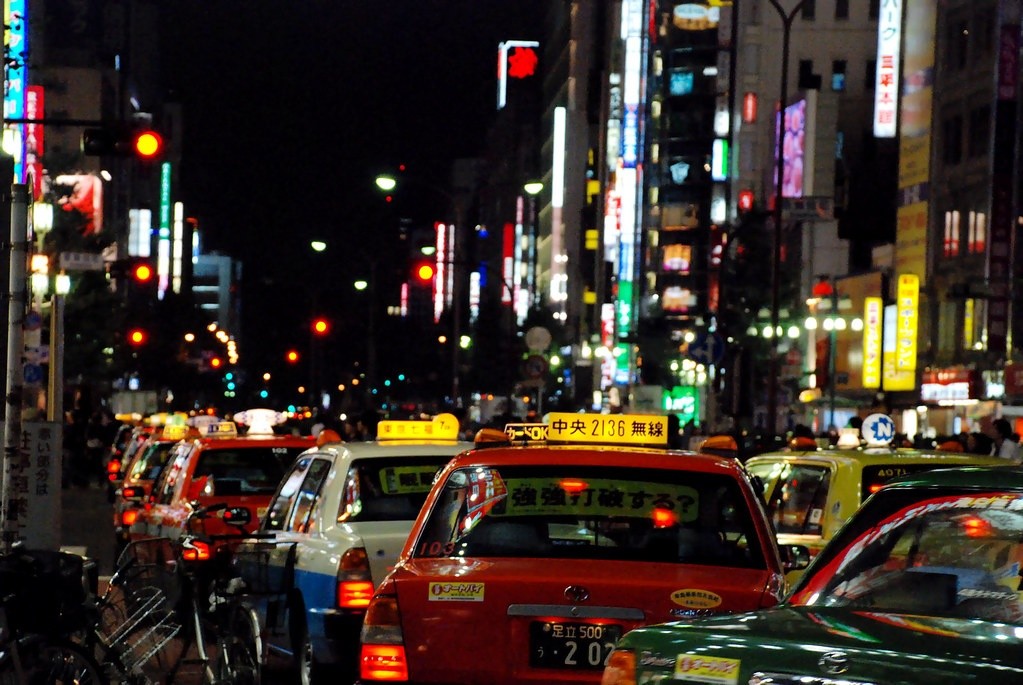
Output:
[82,128,167,162]
[129,327,147,346]
[133,264,152,283]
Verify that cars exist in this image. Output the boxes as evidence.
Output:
[216,408,492,685]
[597,491,1022,684]
[351,410,814,685]
[787,463,1022,615]
[110,405,347,631]
[715,441,1022,607]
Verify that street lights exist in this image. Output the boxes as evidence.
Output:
[373,174,463,407]
[310,235,399,388]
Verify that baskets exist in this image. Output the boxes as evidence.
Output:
[217,543,298,599]
[91,587,181,669]
[116,536,181,624]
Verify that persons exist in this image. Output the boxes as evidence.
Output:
[667,413,1023,459]
[311,407,542,445]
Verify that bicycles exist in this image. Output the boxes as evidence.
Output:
[117,500,263,685]
[0,542,185,685]
